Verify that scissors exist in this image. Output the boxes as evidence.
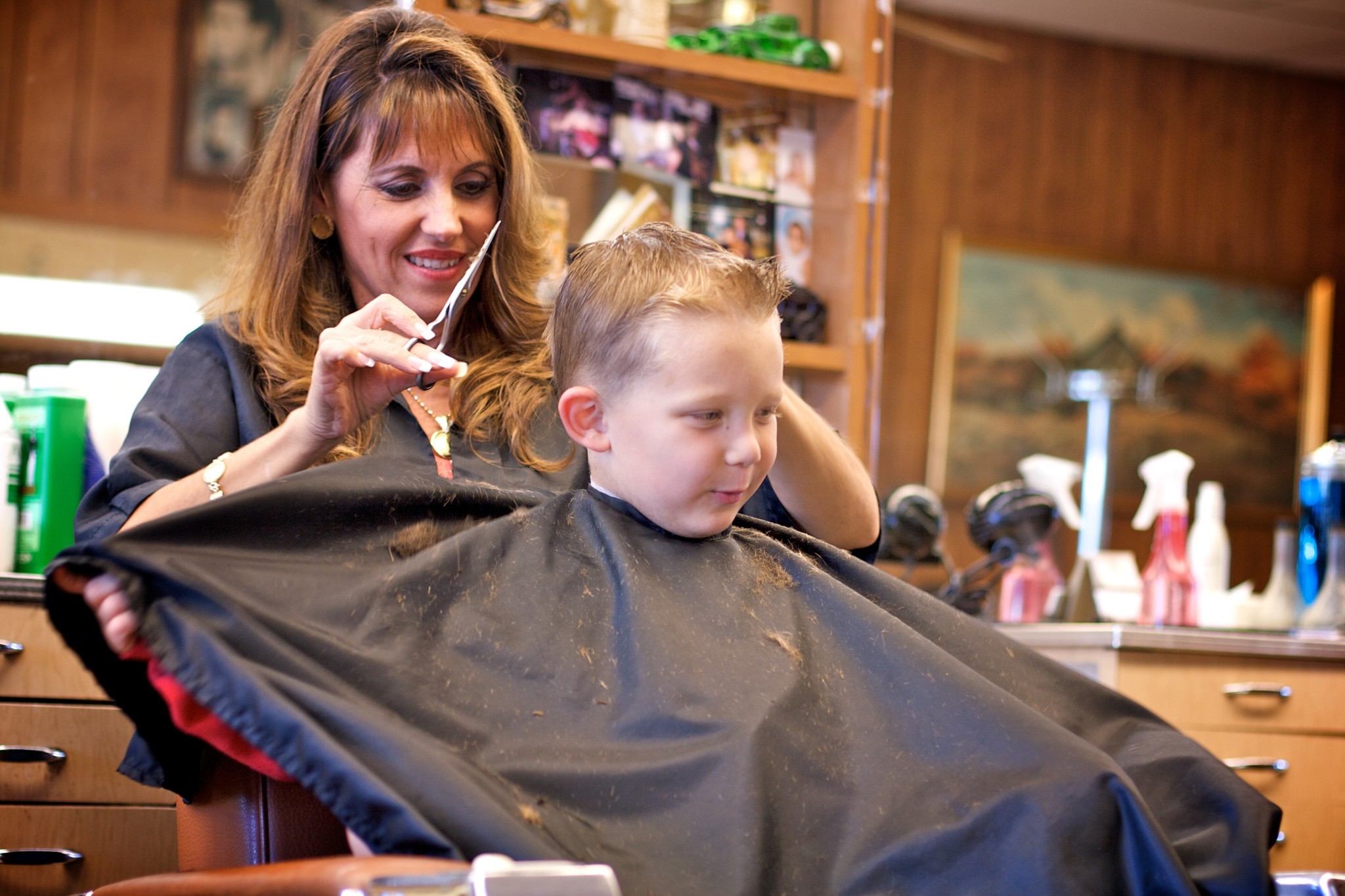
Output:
[401,220,502,390]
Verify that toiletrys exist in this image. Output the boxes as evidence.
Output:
[998,454,1090,626]
[1131,447,1196,627]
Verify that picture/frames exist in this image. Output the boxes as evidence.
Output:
[170,0,386,188]
[921,228,1303,529]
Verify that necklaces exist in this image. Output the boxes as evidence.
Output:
[405,386,452,459]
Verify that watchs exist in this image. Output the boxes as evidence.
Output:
[202,452,235,501]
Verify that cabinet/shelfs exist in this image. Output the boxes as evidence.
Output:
[398,0,897,484]
[1113,651,1344,877]
[0,601,181,896]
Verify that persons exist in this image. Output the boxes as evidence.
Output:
[552,93,812,284]
[72,7,879,566]
[55,225,784,856]
